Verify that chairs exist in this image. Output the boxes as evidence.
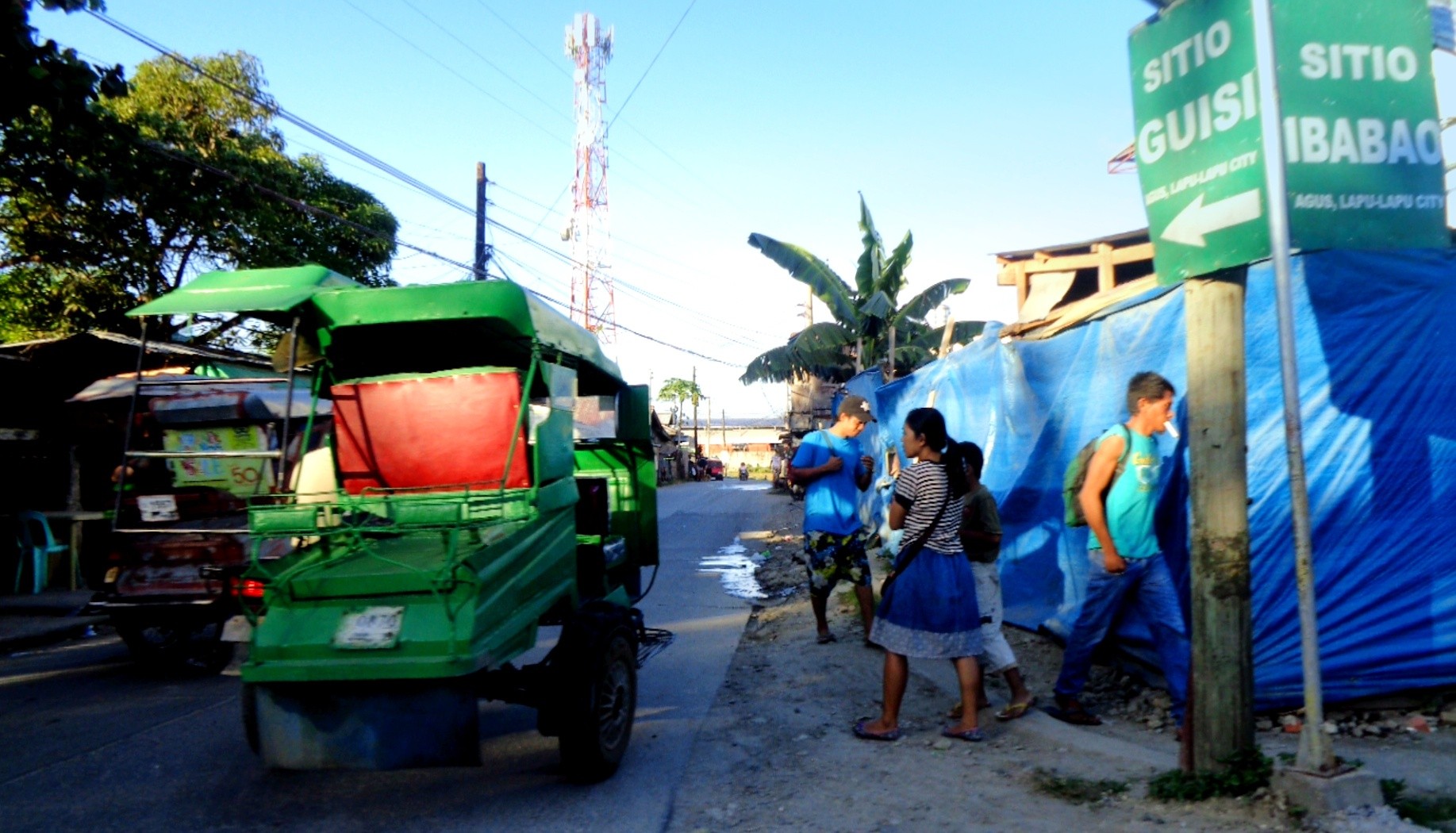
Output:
[9,510,86,597]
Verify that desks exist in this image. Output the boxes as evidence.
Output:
[41,510,116,593]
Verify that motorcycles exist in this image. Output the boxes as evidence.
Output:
[739,471,748,480]
[220,281,676,791]
[706,458,724,481]
[87,263,371,679]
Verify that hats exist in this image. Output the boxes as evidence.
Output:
[842,396,877,422]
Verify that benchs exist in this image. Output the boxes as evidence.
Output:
[572,478,627,600]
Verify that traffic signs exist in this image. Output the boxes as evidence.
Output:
[1129,0,1275,288]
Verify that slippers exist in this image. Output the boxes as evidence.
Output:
[995,696,1036,720]
[946,701,991,718]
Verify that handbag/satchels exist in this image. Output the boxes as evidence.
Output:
[881,569,895,596]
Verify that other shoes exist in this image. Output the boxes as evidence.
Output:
[853,717,899,739]
[1054,690,1101,725]
[942,727,983,742]
[817,633,836,643]
[861,638,882,650]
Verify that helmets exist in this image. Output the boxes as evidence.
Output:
[112,465,135,481]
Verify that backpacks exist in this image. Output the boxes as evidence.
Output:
[1063,423,1131,526]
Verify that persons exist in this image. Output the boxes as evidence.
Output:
[853,408,986,744]
[1048,372,1189,741]
[790,394,883,649]
[946,442,1034,720]
[685,448,785,484]
